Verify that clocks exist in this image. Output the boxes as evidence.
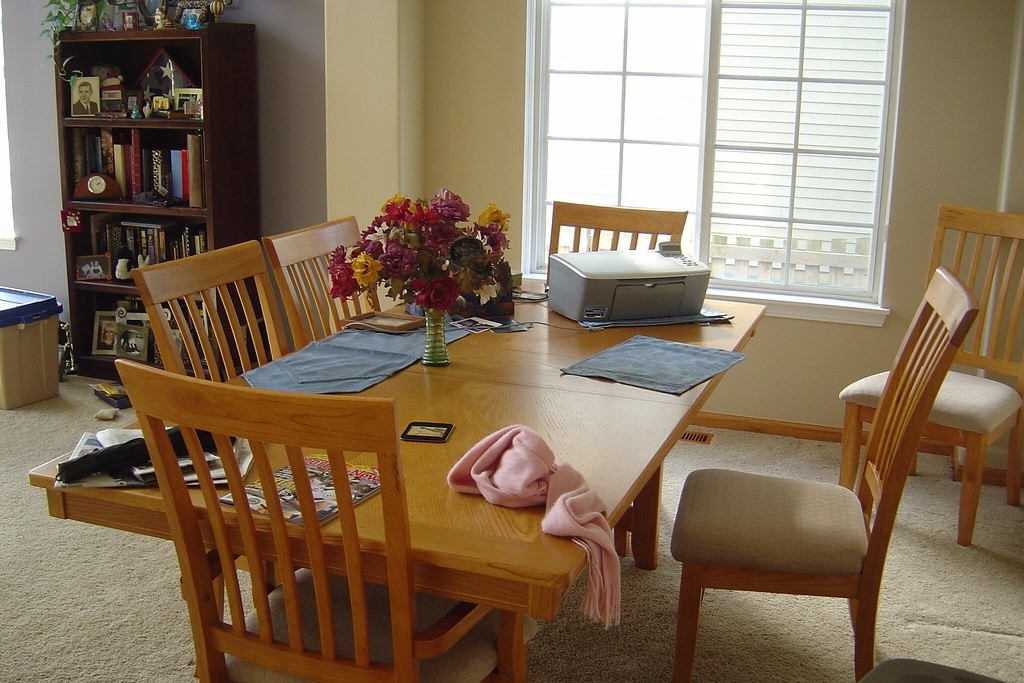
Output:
[72,173,122,199]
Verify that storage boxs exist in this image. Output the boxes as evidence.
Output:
[0,286,65,415]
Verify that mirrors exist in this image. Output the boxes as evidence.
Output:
[137,0,167,30]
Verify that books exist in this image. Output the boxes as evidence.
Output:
[93,381,132,410]
[74,127,204,208]
[90,212,208,274]
[96,426,222,483]
[218,454,382,528]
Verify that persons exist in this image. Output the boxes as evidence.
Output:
[75,1,97,31]
[85,260,106,278]
[73,82,98,115]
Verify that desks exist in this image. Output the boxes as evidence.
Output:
[27,279,768,683]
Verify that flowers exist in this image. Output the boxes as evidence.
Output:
[325,186,512,313]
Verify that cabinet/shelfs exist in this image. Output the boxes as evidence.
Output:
[49,23,263,389]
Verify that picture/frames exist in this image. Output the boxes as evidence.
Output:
[74,254,111,282]
[173,2,209,30]
[72,0,101,32]
[153,319,195,371]
[399,420,457,444]
[114,322,149,363]
[92,310,116,356]
[70,77,100,116]
[115,306,172,328]
[336,311,426,335]
[174,87,203,113]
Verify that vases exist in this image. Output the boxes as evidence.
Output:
[419,307,452,368]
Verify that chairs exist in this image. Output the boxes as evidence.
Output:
[130,236,294,622]
[841,204,1024,550]
[664,262,979,683]
[544,199,692,559]
[110,353,539,683]
[264,214,383,349]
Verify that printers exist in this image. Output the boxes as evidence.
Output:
[548,241,711,322]
